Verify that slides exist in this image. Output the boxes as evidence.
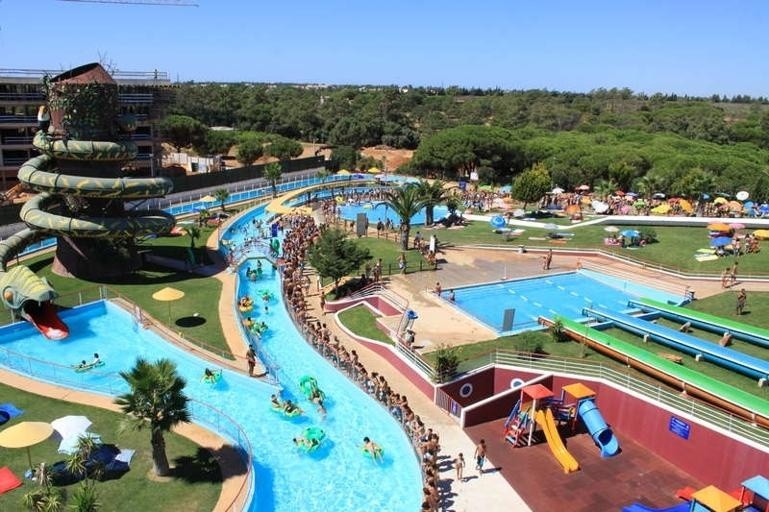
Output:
[536,408,579,474]
[623,486,769,512]
[539,298,768,425]
[576,398,619,458]
[0,128,176,340]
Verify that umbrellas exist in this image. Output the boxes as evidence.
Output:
[0,420,55,467]
[48,415,93,440]
[0,403,25,425]
[57,433,105,454]
[200,194,217,203]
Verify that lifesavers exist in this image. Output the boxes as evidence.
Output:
[361,444,382,459]
[300,377,316,392]
[238,297,253,313]
[204,372,221,384]
[248,269,260,277]
[74,360,104,373]
[273,239,279,250]
[257,288,274,301]
[273,401,299,418]
[244,319,268,336]
[308,390,325,404]
[299,428,324,451]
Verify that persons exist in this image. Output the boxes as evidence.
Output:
[228,216,267,283]
[542,256,546,270]
[736,288,746,315]
[289,287,370,392]
[350,217,441,288]
[433,282,442,296]
[270,392,384,468]
[740,293,746,314]
[717,234,761,258]
[504,212,512,226]
[246,344,257,377]
[88,352,100,365]
[538,191,582,209]
[261,188,266,195]
[277,198,342,300]
[400,330,415,346]
[200,367,219,383]
[449,288,456,301]
[237,296,272,338]
[547,248,552,269]
[474,439,487,474]
[371,371,440,510]
[721,267,731,287]
[457,190,507,211]
[719,330,732,347]
[313,186,393,206]
[455,453,465,480]
[544,230,649,247]
[67,360,87,369]
[728,261,738,285]
